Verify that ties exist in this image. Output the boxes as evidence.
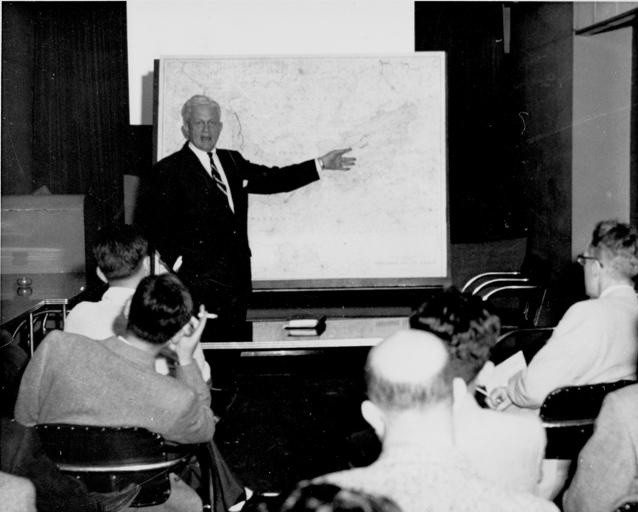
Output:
[208,152,228,202]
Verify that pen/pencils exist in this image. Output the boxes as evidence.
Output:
[199,310,220,320]
[475,387,502,404]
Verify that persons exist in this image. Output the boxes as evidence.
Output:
[138,94,355,343]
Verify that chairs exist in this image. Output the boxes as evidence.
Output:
[615,495,638,511]
[461,237,589,365]
[24,420,199,511]
[539,380,638,471]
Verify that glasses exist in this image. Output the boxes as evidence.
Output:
[576,254,602,270]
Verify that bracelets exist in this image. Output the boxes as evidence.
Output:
[317,157,325,169]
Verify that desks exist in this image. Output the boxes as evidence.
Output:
[183,317,414,486]
[0,270,95,409]
